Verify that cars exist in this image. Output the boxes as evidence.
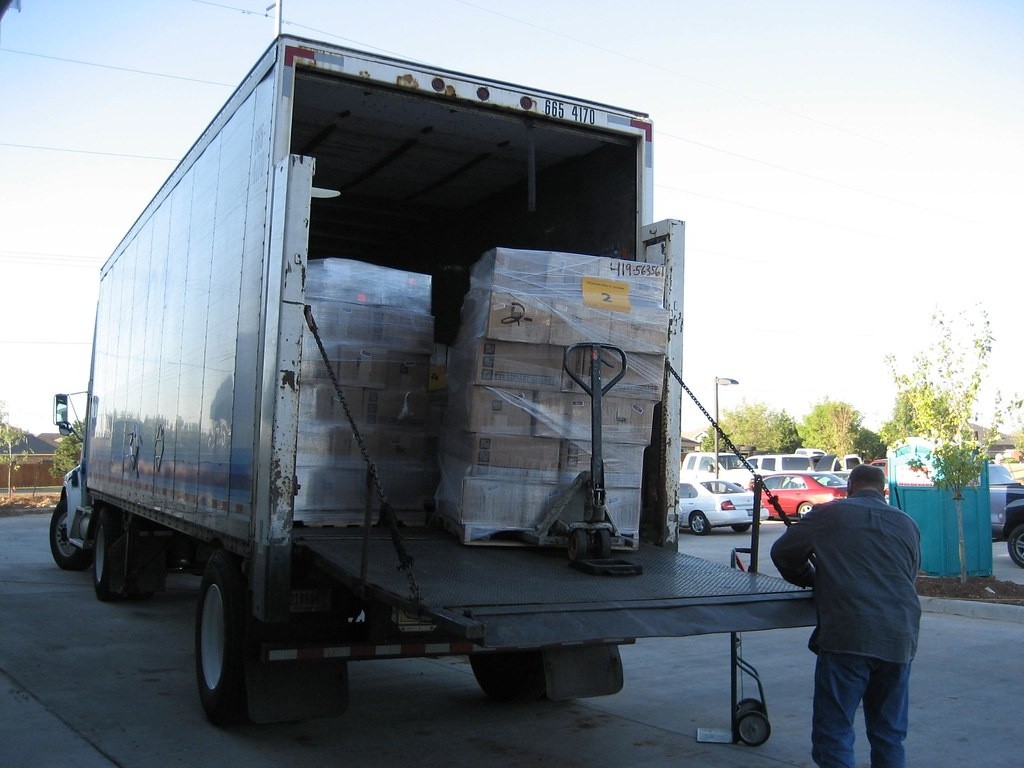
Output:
[985,455,1024,567]
[678,475,770,536]
[686,446,889,504]
[743,473,852,520]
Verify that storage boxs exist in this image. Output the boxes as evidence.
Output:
[291,244,672,533]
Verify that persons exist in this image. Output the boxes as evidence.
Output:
[769,463,922,768]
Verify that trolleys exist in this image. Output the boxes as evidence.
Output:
[694,474,774,746]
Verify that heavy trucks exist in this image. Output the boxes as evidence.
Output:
[44,31,823,730]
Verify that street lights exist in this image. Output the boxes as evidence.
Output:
[713,374,739,480]
